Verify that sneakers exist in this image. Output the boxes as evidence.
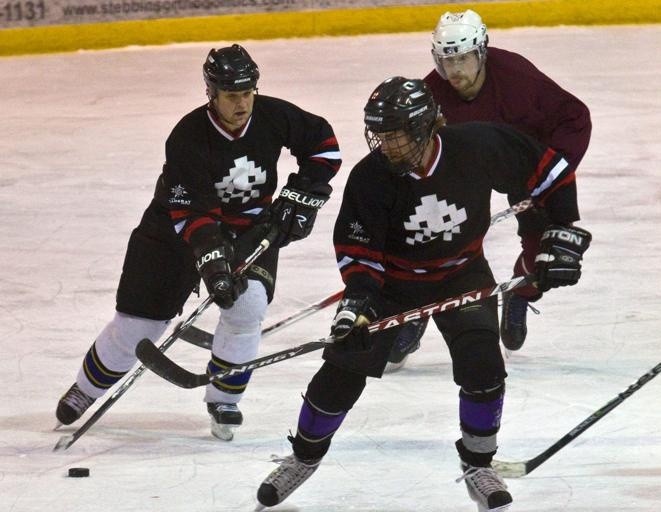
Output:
[456,454,513,510]
[257,447,321,506]
[501,292,540,350]
[207,392,242,424]
[390,321,427,362]
[57,380,97,425]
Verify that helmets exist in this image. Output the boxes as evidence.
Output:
[363,76,436,176]
[204,44,259,97]
[431,10,488,89]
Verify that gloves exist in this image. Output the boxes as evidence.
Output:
[192,238,248,309]
[254,173,332,248]
[535,225,592,292]
[324,293,384,355]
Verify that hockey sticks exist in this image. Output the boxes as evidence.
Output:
[135,273,540,389]
[52,224,280,452]
[489,363,661,479]
[174,199,533,350]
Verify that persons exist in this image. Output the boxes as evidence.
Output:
[56,44,343,428]
[386,9,592,365]
[256,77,590,509]
